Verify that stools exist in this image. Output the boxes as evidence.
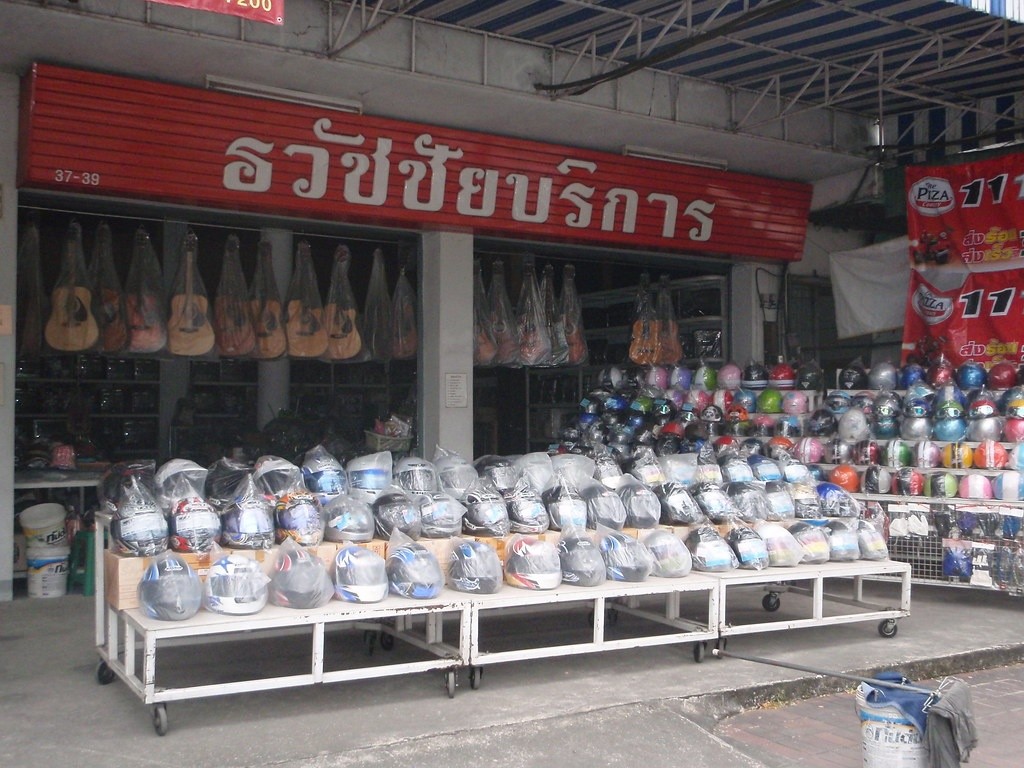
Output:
[68,530,108,596]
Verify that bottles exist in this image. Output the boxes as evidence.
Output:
[65,506,80,541]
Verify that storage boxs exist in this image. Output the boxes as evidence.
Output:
[337,538,386,560]
[181,549,232,582]
[233,545,280,573]
[539,522,788,543]
[474,533,538,580]
[103,549,167,610]
[417,534,475,585]
[364,428,414,452]
[13,533,27,572]
[303,540,337,576]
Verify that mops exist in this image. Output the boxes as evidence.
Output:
[712,649,978,768]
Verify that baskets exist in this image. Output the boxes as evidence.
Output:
[364,430,414,451]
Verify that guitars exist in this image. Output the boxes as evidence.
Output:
[325,243,362,359]
[46,221,99,352]
[248,235,291,360]
[473,256,590,366]
[367,248,418,362]
[88,224,132,353]
[164,231,215,355]
[284,240,329,358]
[123,228,169,353]
[211,232,256,357]
[630,273,685,368]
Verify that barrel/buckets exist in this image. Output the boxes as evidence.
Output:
[26,547,71,599]
[19,503,69,546]
[854,684,924,768]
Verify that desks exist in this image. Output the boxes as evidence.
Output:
[14,472,104,508]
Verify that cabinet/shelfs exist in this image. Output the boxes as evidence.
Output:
[189,357,259,455]
[290,359,416,432]
[15,354,160,456]
[526,277,1024,509]
[94,511,470,736]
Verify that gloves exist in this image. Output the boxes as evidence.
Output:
[885,502,1024,589]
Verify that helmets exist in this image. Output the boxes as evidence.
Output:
[419,496,463,540]
[372,493,422,540]
[138,555,202,620]
[220,495,273,551]
[268,548,335,609]
[345,455,392,504]
[170,497,222,555]
[388,543,443,600]
[438,453,890,590]
[546,359,1024,503]
[102,461,155,513]
[274,491,324,548]
[205,457,254,510]
[200,553,270,616]
[446,541,503,595]
[301,456,348,505]
[253,456,305,506]
[110,495,166,558]
[393,456,439,499]
[321,496,375,543]
[153,458,210,513]
[333,546,388,603]
